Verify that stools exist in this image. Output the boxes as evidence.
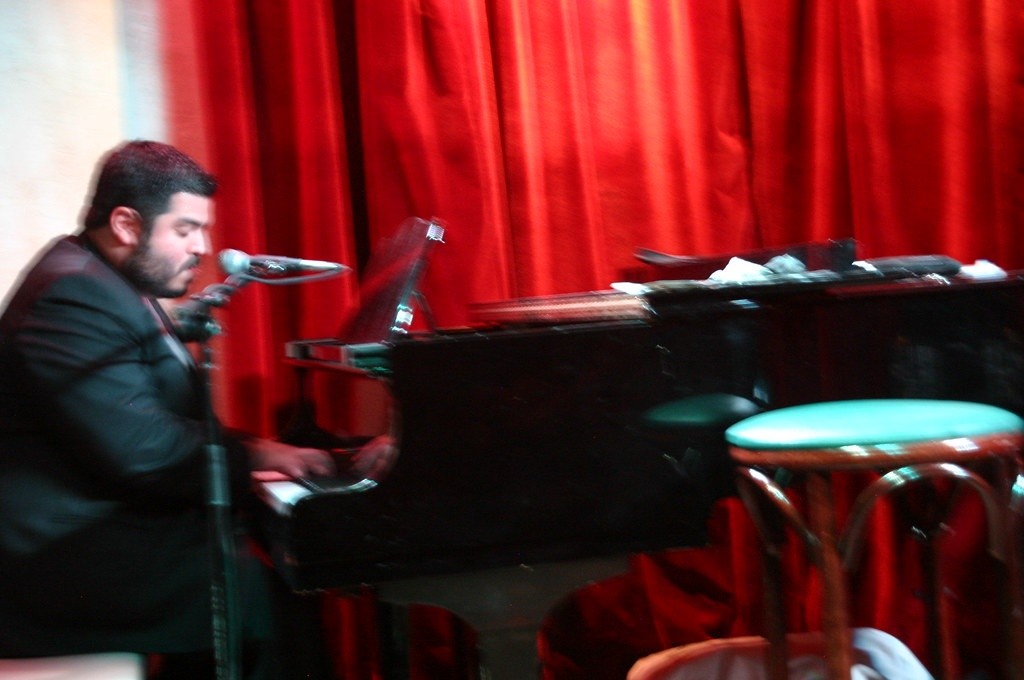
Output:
[633,392,756,544]
[726,400,1022,680]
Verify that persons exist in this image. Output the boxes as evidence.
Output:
[0,139,340,680]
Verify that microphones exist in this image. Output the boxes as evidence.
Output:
[218,248,350,276]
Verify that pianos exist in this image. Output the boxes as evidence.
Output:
[232,239,1023,680]
